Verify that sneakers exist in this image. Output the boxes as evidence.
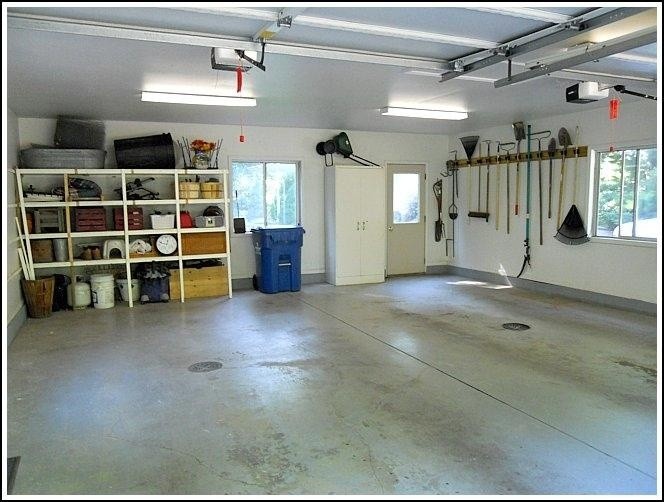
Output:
[79,247,102,260]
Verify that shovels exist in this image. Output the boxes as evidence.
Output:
[513,122,525,215]
[557,126,572,232]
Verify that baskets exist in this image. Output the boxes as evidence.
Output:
[21,275,55,319]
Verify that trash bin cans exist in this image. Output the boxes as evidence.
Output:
[253,227,304,291]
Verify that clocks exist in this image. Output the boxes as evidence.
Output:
[155,235,178,255]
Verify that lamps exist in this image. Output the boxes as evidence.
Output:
[140,92,257,108]
[380,106,468,121]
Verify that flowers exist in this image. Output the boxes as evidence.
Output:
[190,139,215,155]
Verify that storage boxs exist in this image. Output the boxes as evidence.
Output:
[180,232,226,254]
[113,207,144,229]
[34,208,74,234]
[169,265,229,300]
[71,208,107,232]
[149,214,175,229]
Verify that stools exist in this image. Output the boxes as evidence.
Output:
[102,239,126,259]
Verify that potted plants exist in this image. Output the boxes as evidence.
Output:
[200,177,222,199]
[179,175,199,199]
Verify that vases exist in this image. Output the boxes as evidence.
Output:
[193,153,209,170]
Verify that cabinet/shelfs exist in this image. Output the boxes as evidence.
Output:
[16,167,232,310]
[325,166,387,286]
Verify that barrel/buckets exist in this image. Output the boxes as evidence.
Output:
[179,182,200,199]
[90,274,116,309]
[200,181,223,199]
